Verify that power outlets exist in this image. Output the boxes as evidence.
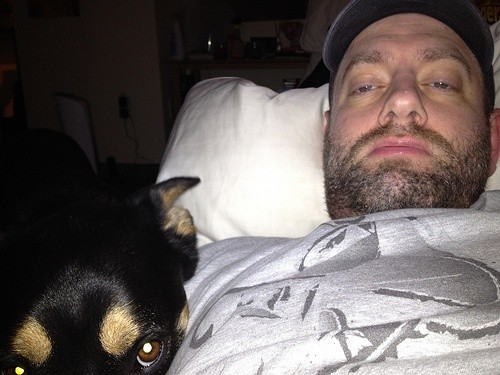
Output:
[113,93,141,111]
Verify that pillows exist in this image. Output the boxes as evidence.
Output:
[156,77,499,242]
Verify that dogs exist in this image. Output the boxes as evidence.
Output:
[0,176,201,375]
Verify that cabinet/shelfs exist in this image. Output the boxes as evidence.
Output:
[156,2,314,142]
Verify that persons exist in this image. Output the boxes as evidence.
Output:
[162,0,500,375]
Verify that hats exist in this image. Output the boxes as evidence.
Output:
[322,1,493,74]
